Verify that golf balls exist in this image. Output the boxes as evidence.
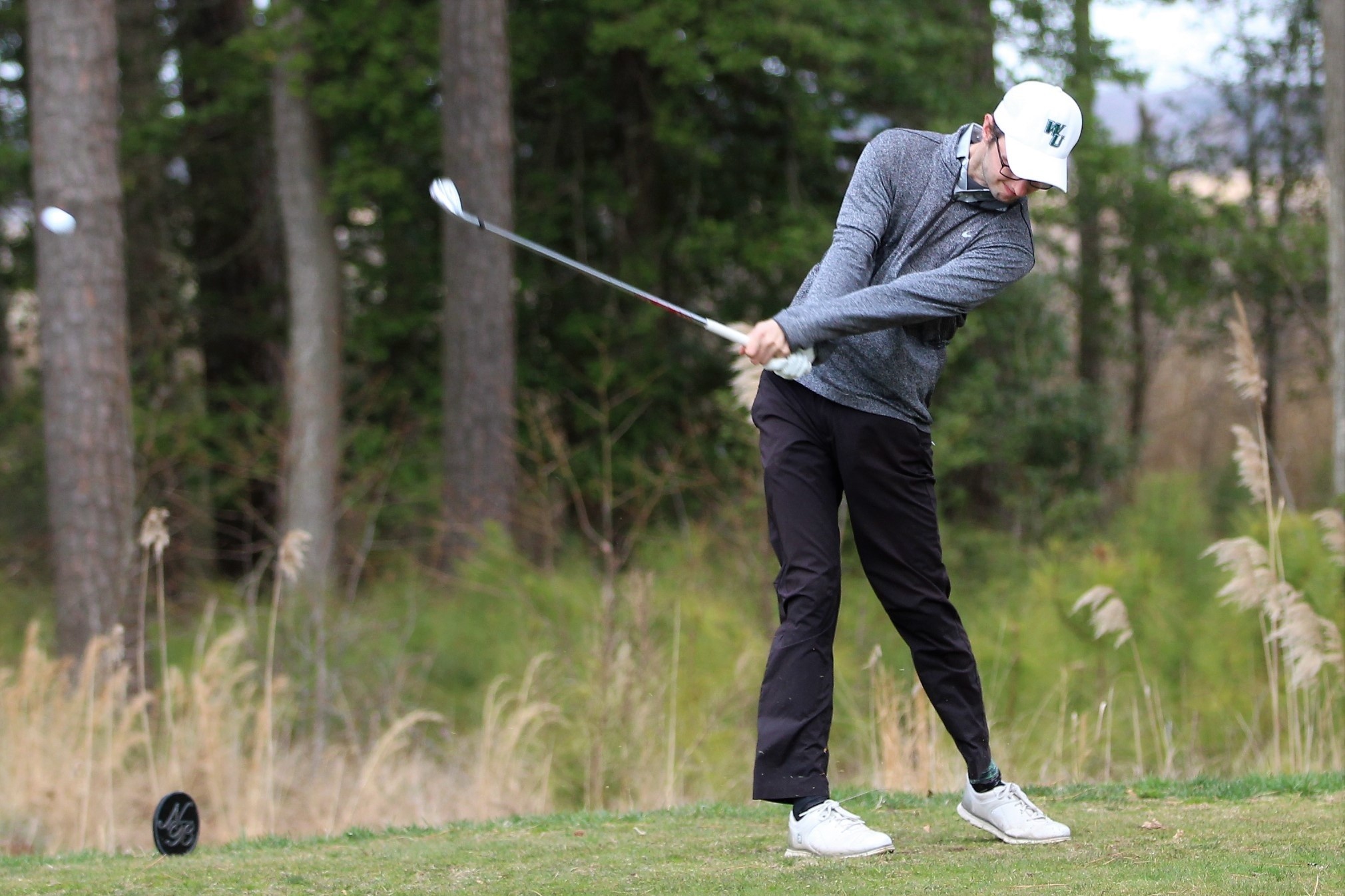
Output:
[39,205,77,233]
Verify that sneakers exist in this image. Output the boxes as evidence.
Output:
[784,799,895,857]
[957,772,1071,844]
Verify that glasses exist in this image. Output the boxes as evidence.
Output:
[996,137,1053,190]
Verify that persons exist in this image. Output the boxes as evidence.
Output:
[739,79,1083,858]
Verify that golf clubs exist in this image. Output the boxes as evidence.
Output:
[426,175,751,347]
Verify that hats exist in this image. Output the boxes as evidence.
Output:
[993,80,1082,194]
